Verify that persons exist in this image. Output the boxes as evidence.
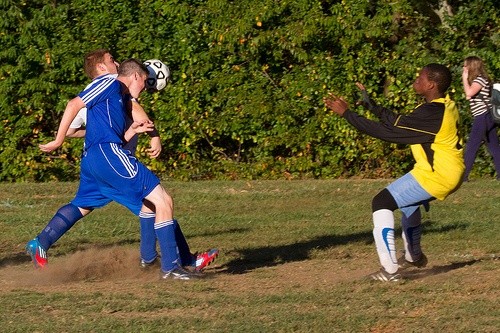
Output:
[322,63,466,284]
[462,56,500,181]
[37,58,191,281]
[25,49,219,272]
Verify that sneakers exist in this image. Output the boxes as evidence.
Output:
[140,257,162,273]
[183,247,219,274]
[159,265,192,281]
[364,266,401,282]
[26,237,48,273]
[398,252,427,270]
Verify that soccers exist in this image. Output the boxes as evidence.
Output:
[142,58,171,93]
